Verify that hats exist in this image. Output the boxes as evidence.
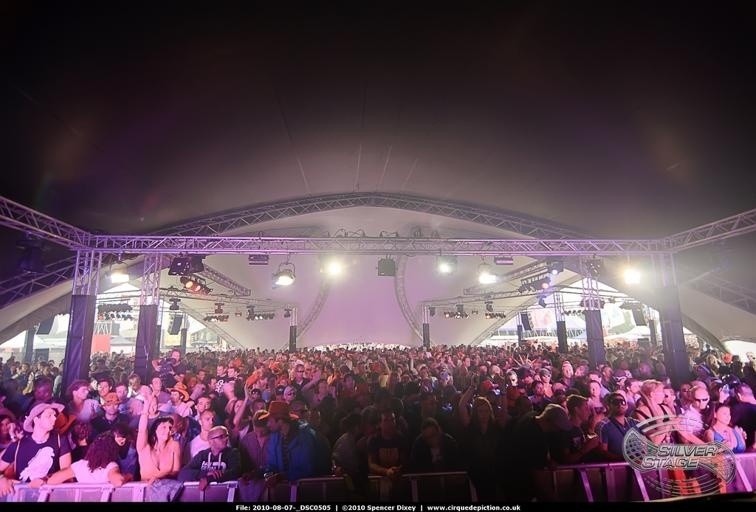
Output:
[22,402,65,433]
[545,403,572,432]
[479,380,498,392]
[54,413,76,435]
[166,382,189,402]
[257,401,299,422]
[99,393,122,406]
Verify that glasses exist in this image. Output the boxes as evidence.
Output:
[613,399,626,406]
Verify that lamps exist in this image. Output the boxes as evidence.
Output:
[14,236,46,274]
[429,253,646,320]
[95,254,298,323]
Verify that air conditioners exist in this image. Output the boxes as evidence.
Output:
[374,252,400,278]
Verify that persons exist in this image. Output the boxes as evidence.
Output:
[0,338,756,500]
